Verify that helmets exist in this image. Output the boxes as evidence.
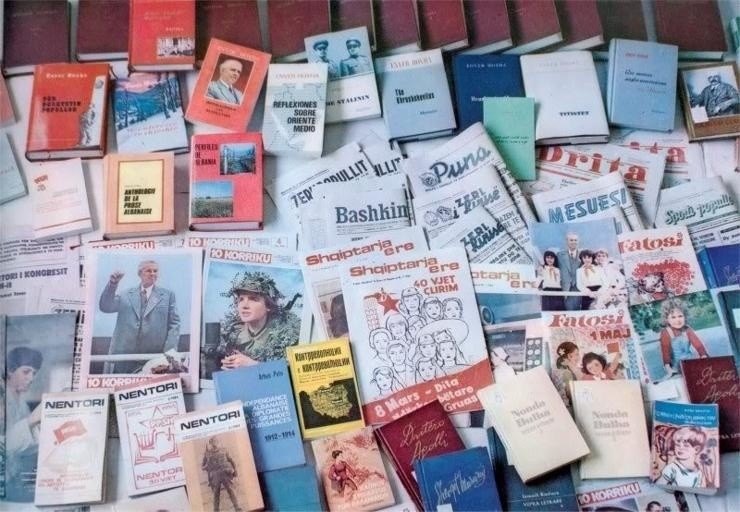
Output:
[232,276,277,296]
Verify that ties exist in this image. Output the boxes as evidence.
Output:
[141,290,146,313]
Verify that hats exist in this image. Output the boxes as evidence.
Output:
[313,41,327,50]
[347,39,360,47]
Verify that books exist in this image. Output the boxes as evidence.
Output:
[0,0,740,512]
